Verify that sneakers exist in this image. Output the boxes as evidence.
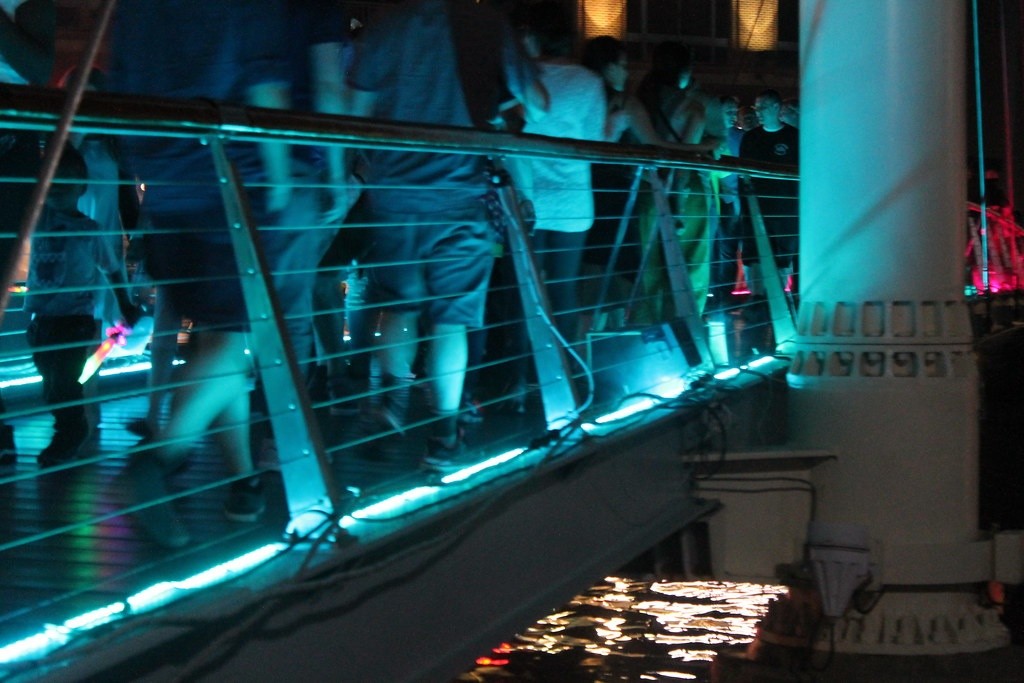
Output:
[330,394,361,415]
[38,412,91,464]
[420,440,496,472]
[459,400,485,422]
[225,479,266,522]
[375,394,408,436]
[116,475,190,548]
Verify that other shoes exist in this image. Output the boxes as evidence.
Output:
[506,400,527,413]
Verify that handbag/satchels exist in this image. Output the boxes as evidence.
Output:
[479,156,535,237]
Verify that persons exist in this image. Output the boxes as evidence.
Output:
[0,0,800,548]
[965,167,1009,295]
